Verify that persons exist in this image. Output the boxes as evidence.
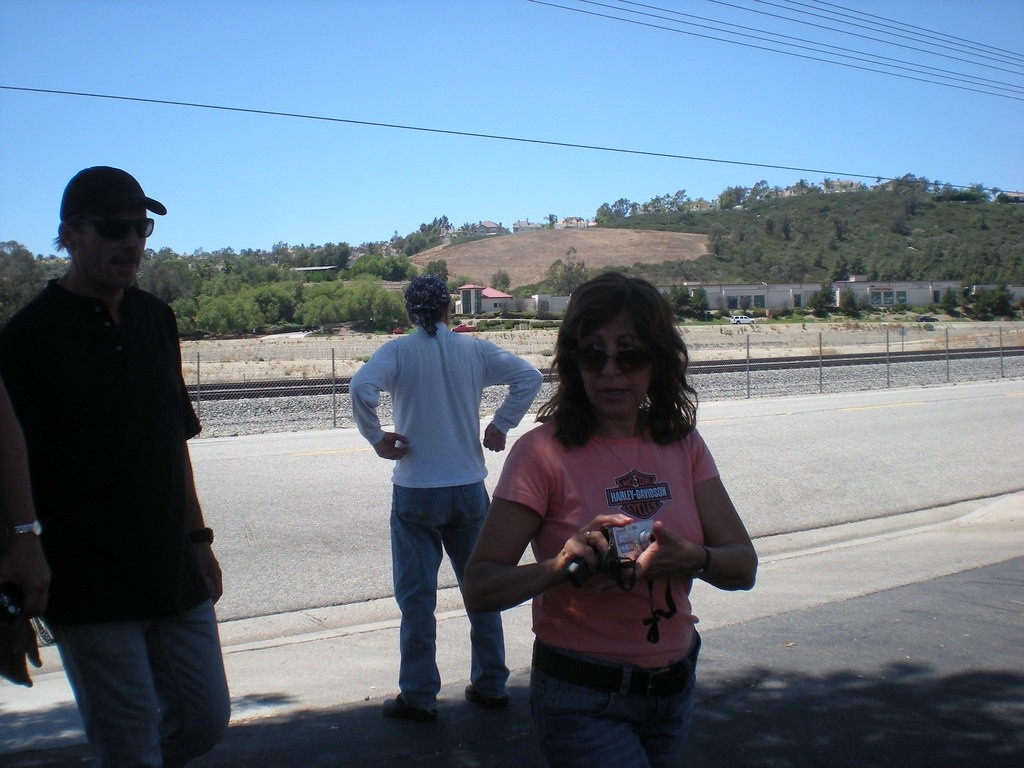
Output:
[463,272,758,768]
[349,276,541,718]
[0,165,232,768]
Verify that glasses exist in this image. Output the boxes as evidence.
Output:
[73,216,154,240]
[568,345,657,372]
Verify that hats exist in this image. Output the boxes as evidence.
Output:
[60,166,167,219]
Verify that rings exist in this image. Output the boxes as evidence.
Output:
[585,532,590,543]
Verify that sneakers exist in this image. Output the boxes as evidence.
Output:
[464,684,512,706]
[383,694,438,721]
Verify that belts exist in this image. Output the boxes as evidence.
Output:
[533,629,703,699]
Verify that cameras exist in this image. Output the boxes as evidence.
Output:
[607,517,657,566]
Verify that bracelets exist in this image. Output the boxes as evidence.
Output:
[683,545,711,579]
[184,528,214,546]
[5,520,42,536]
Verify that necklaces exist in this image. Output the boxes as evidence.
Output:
[601,435,641,488]
